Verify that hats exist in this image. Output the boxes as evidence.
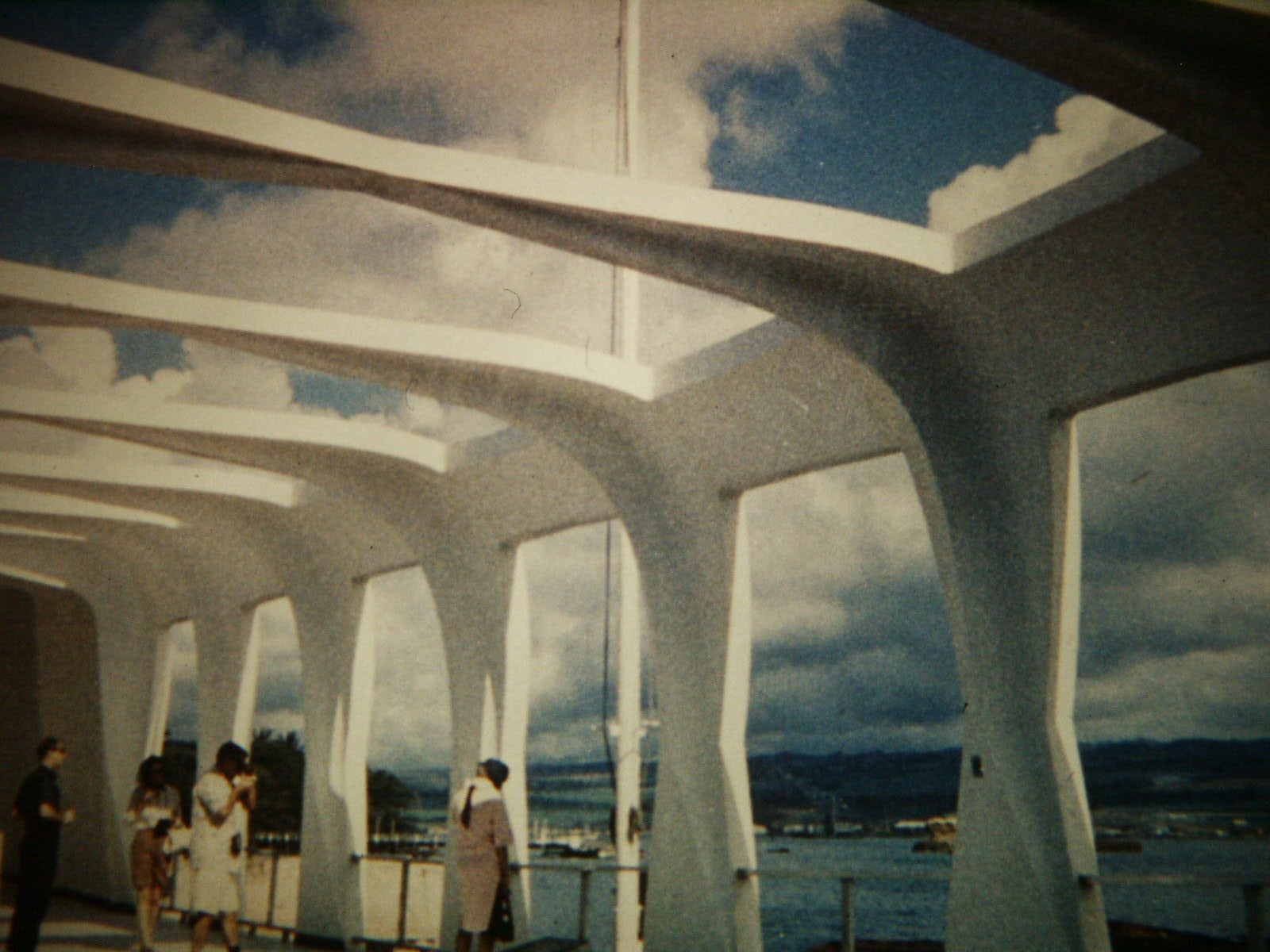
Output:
[481,759,508,791]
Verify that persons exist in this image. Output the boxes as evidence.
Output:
[449,760,515,952]
[6,733,77,952]
[187,742,258,952]
[122,755,183,952]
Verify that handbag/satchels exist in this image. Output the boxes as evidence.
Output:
[490,881,514,940]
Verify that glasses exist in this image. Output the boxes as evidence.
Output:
[53,747,65,755]
